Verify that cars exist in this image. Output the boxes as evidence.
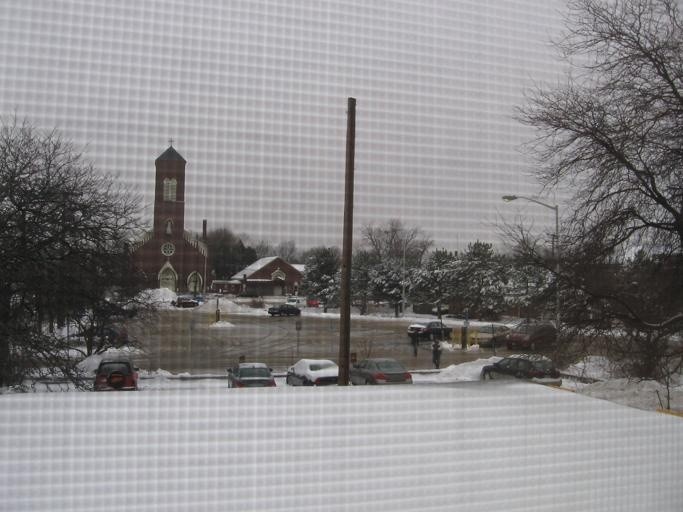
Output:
[407,321,453,342]
[171,295,199,308]
[350,358,413,384]
[227,362,277,388]
[467,320,557,351]
[268,298,306,316]
[480,354,562,387]
[287,357,341,386]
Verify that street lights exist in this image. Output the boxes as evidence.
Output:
[502,195,560,334]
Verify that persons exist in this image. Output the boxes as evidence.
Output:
[410,330,419,356]
[430,338,442,369]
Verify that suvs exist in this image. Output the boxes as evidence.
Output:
[93,356,139,392]
